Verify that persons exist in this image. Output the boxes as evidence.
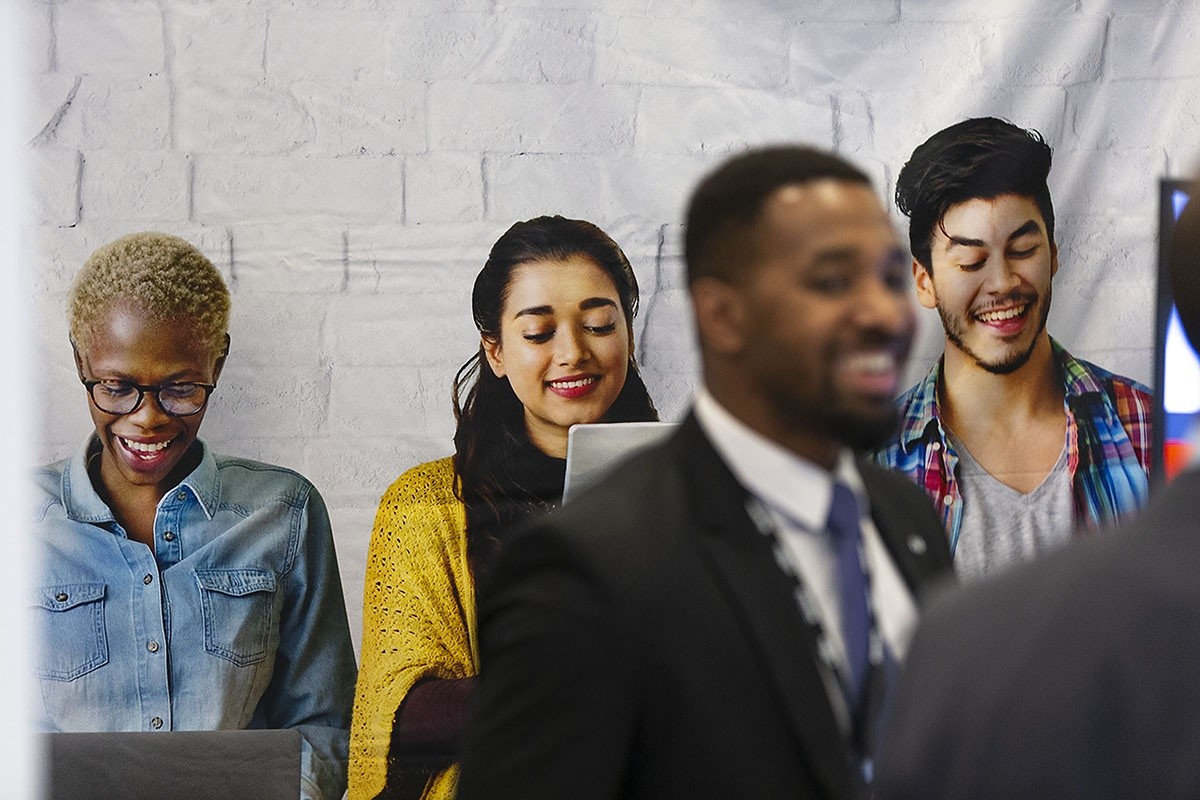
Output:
[862,118,1160,594]
[344,215,663,800]
[0,232,359,800]
[455,144,958,800]
[880,190,1200,800]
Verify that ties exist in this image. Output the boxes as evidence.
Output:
[832,484,872,710]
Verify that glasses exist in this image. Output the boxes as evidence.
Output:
[82,377,217,416]
